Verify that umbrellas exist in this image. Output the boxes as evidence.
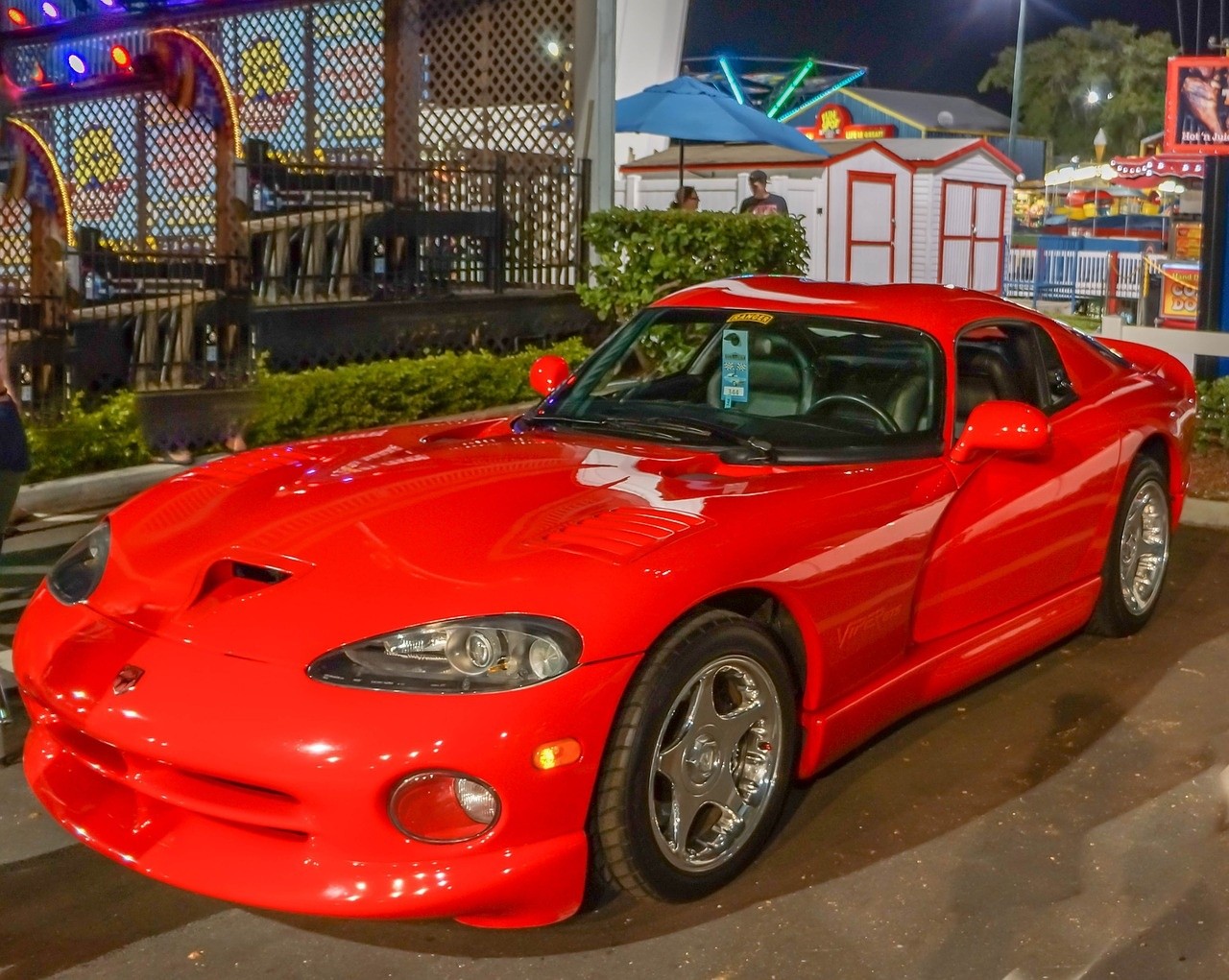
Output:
[537,66,831,210]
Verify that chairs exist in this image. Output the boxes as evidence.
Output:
[877,345,1019,439]
[705,330,824,418]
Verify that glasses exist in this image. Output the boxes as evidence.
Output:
[688,196,699,202]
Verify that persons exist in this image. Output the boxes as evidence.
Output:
[740,170,788,216]
[0,383,31,544]
[669,186,699,209]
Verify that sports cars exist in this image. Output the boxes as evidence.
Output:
[12,280,1194,928]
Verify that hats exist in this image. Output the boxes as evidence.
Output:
[749,170,769,181]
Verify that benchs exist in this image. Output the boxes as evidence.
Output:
[813,339,1013,433]
